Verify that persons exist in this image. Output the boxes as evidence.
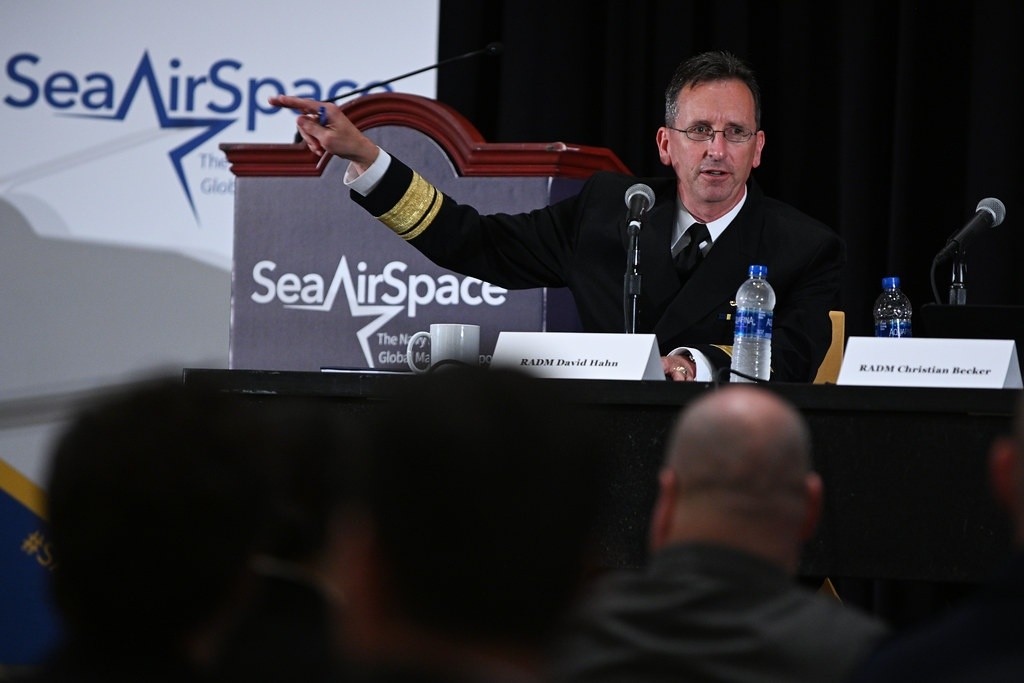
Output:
[8,365,600,683]
[568,388,889,683]
[269,54,850,385]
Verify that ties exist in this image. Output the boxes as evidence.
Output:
[673,223,713,267]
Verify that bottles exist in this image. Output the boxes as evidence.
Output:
[874,277,913,338]
[730,265,776,384]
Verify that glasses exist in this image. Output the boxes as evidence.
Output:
[668,125,757,142]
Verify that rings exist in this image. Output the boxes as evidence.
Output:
[675,366,687,375]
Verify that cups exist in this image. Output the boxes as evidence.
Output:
[405,323,481,375]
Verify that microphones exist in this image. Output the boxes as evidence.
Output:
[625,183,654,237]
[936,198,1006,261]
[326,41,504,102]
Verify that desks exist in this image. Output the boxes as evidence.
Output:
[181,369,1024,632]
